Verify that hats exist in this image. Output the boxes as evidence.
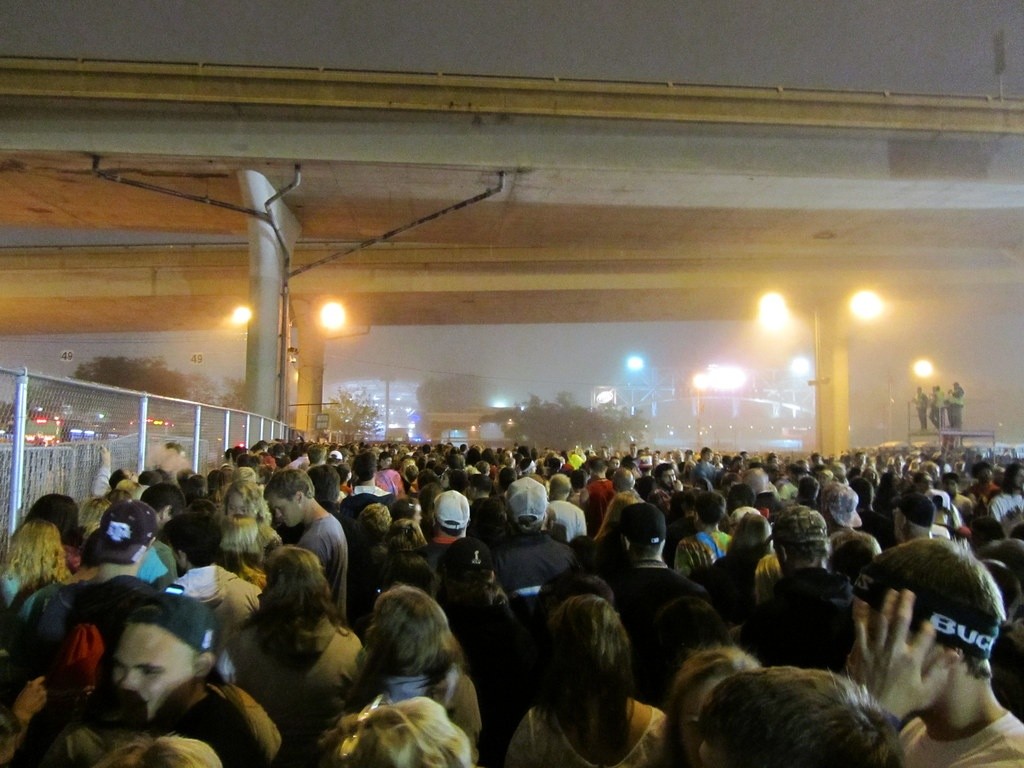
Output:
[340,447,353,456]
[79,499,158,563]
[822,481,863,528]
[131,592,230,686]
[620,502,667,544]
[329,451,343,460]
[924,488,951,512]
[434,490,470,529]
[504,476,548,525]
[445,536,492,579]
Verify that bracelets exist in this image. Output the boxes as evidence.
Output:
[880,708,901,731]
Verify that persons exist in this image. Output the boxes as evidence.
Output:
[0,431,1024,768]
[912,380,962,429]
